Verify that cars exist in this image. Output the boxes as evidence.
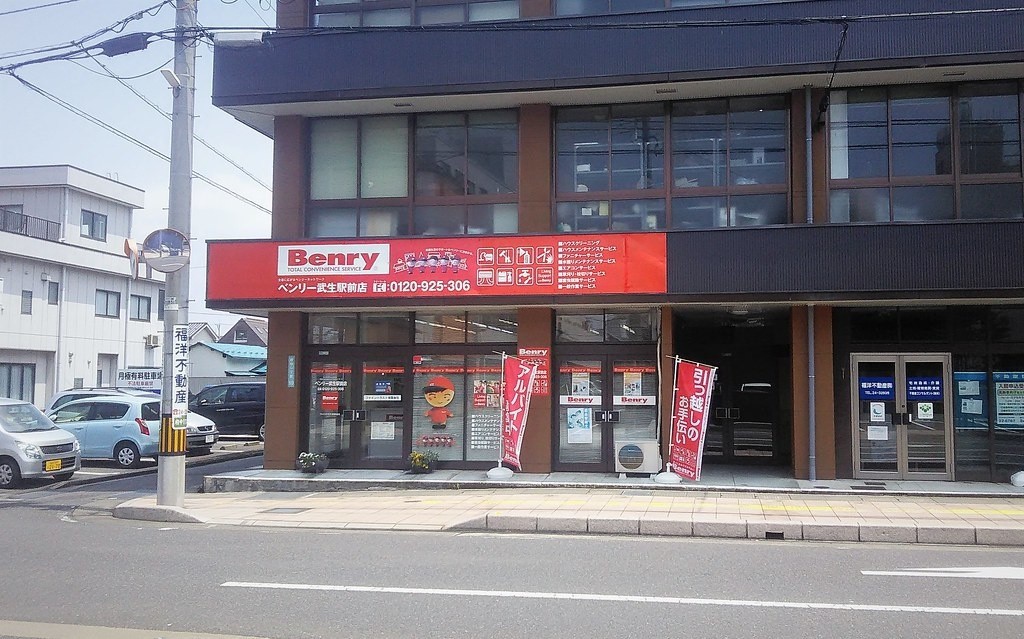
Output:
[0,398,81,489]
[46,397,161,468]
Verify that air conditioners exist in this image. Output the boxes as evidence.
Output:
[615,441,663,474]
[147,334,159,346]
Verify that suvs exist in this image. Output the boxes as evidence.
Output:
[43,386,219,456]
[189,382,266,441]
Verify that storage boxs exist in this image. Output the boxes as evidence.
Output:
[493,203,518,233]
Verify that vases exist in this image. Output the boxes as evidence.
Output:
[296,458,330,473]
[414,462,433,474]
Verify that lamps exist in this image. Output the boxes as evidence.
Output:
[88,360,91,364]
[41,273,52,281]
[68,352,74,362]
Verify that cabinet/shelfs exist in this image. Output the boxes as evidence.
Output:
[859,414,1024,473]
[716,135,786,225]
[703,422,773,456]
[645,138,716,230]
[573,142,644,232]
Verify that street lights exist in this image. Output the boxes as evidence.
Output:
[158,70,195,516]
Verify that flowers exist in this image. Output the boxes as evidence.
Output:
[298,452,328,469]
[410,449,440,469]
